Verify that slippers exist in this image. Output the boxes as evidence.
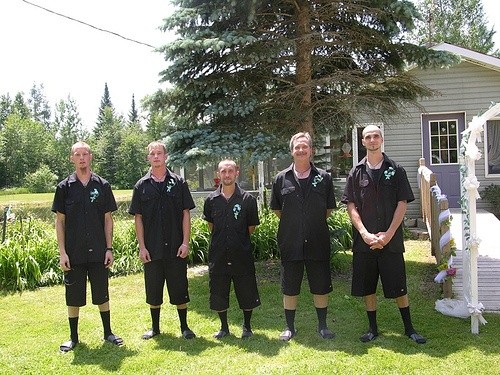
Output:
[103,335,123,344]
[404,332,426,344]
[242,331,253,338]
[60,340,75,352]
[181,328,196,339]
[216,329,230,337]
[317,328,335,339]
[142,331,160,339]
[359,332,379,342]
[280,328,298,340]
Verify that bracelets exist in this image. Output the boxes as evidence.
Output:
[182,243,190,248]
[106,248,113,252]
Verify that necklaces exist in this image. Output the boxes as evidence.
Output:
[151,170,168,183]
[295,165,311,177]
[368,157,384,170]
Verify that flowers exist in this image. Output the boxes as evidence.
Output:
[445,266,457,285]
[457,100,500,252]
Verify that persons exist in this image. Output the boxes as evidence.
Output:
[128,141,196,341]
[51,141,124,353]
[269,132,337,341]
[341,125,427,344]
[201,160,261,340]
